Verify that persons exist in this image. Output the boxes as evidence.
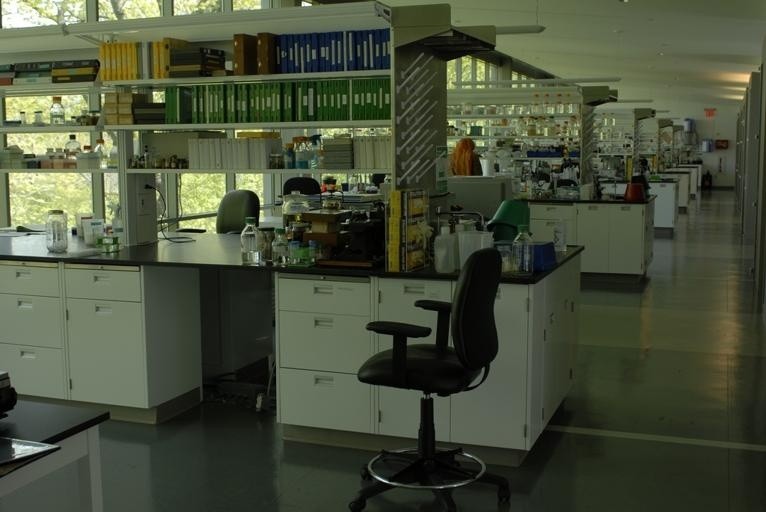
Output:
[448,138,483,176]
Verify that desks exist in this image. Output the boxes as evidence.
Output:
[0,398,112,512]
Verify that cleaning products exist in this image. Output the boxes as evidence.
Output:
[292,136,309,169]
[306,134,322,168]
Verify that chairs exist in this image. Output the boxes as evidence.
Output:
[478,197,534,244]
[174,189,262,236]
[346,247,512,512]
[280,178,323,195]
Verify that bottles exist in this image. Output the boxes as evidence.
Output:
[240,190,316,266]
[269,139,311,169]
[128,145,188,169]
[599,113,632,154]
[47,209,67,252]
[2,134,119,168]
[448,101,580,115]
[491,137,580,158]
[433,223,535,276]
[447,116,580,137]
[648,138,656,154]
[18,96,100,126]
[520,160,578,200]
[349,175,358,194]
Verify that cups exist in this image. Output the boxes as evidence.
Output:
[554,225,567,251]
[76,214,124,253]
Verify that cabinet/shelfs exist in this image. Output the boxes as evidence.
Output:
[538,195,658,277]
[272,244,586,449]
[0,2,451,247]
[0,261,204,408]
[596,102,705,231]
[447,79,619,185]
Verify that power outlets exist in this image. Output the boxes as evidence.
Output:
[135,174,155,195]
